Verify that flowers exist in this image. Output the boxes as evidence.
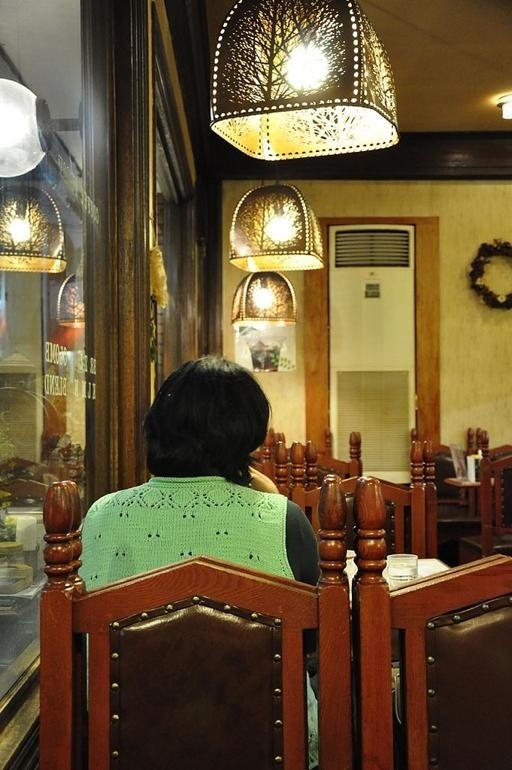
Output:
[470,240,512,311]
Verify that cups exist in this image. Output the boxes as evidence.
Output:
[387,552,419,591]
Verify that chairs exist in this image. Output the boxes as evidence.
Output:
[39,427,512,770]
[0,433,84,506]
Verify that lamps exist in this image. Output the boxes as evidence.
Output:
[210,1,400,160]
[56,271,86,328]
[495,95,512,120]
[229,167,324,273]
[231,272,297,326]
[1,79,82,178]
[1,187,68,273]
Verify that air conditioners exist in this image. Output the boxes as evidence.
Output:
[327,224,417,485]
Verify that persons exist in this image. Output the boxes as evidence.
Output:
[74,354,320,769]
